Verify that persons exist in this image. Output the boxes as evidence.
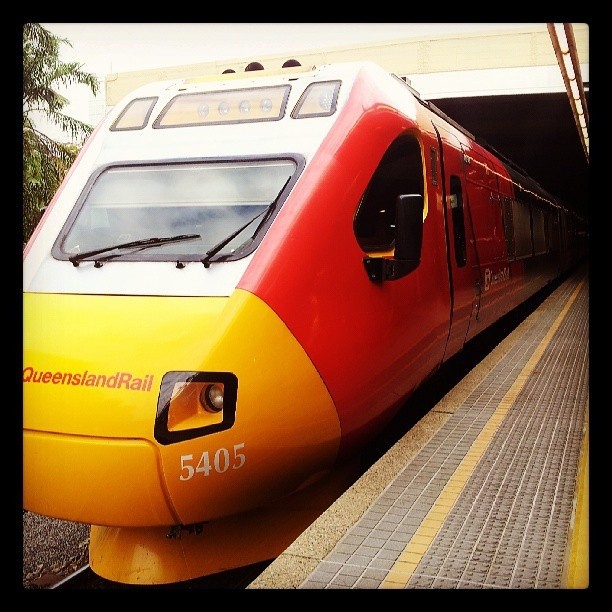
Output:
[366,134,424,242]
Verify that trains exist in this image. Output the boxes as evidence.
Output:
[24,56,591,584]
